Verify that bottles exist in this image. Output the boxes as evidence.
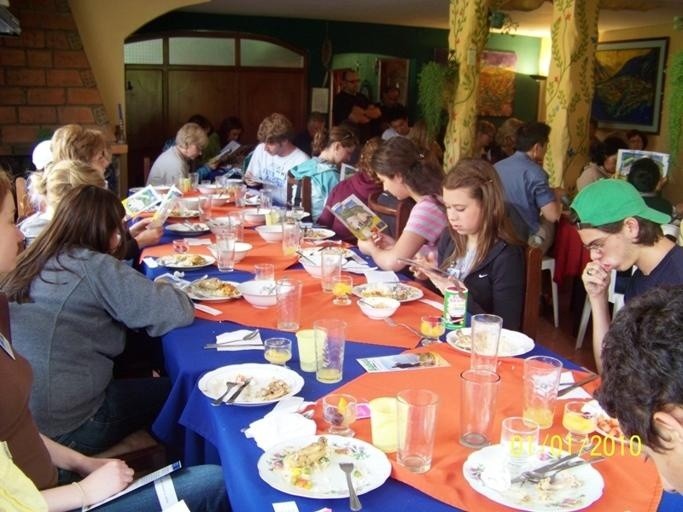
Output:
[444,286,468,330]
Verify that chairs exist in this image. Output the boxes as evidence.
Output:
[539,256,559,330]
[368,191,408,245]
[575,224,680,349]
[286,170,313,222]
[507,240,542,341]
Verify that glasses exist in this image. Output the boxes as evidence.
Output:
[336,131,352,144]
[343,77,361,84]
[581,232,616,250]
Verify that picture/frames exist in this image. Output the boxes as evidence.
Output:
[590,36,670,136]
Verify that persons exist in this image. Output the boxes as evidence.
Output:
[0,168,226,512]
[0,182,195,457]
[381,106,432,154]
[20,159,165,261]
[202,116,243,161]
[374,85,406,134]
[626,156,673,218]
[144,122,221,189]
[625,129,648,152]
[487,118,524,165]
[333,68,381,144]
[575,137,630,195]
[242,113,311,207]
[566,178,683,380]
[162,113,221,184]
[46,123,112,172]
[315,138,388,245]
[293,112,327,157]
[286,125,358,221]
[357,136,451,272]
[491,123,564,257]
[475,120,496,161]
[408,157,524,332]
[591,284,683,512]
[242,117,270,177]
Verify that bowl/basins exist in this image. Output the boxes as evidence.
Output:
[207,241,253,264]
[298,253,347,279]
[225,179,244,187]
[255,224,294,244]
[237,278,291,311]
[356,295,401,320]
[206,194,230,207]
[182,197,202,210]
[207,218,232,234]
[196,185,223,195]
[242,208,272,223]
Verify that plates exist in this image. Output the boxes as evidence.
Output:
[184,280,245,302]
[446,326,536,360]
[302,227,337,241]
[197,362,306,407]
[301,245,355,261]
[243,198,270,206]
[285,210,311,219]
[169,209,203,218]
[462,442,605,512]
[257,433,393,500]
[352,282,426,303]
[165,222,209,237]
[155,253,216,272]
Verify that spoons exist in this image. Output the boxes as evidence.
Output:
[207,328,260,346]
[519,456,606,484]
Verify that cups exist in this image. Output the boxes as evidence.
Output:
[214,233,237,273]
[420,315,447,347]
[312,318,347,386]
[459,367,502,448]
[396,389,439,473]
[320,252,343,294]
[264,337,293,367]
[522,356,563,431]
[274,279,302,333]
[234,187,248,209]
[294,328,327,374]
[470,313,504,376]
[202,179,211,185]
[562,400,600,441]
[291,205,305,227]
[253,264,276,281]
[321,392,358,439]
[188,173,200,193]
[174,240,190,255]
[500,416,540,475]
[215,176,226,185]
[368,396,410,454]
[228,212,244,243]
[281,223,301,257]
[331,274,353,307]
[259,190,273,213]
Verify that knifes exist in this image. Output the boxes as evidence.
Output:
[557,374,598,397]
[204,342,265,351]
[224,379,252,405]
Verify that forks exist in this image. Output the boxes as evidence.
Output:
[338,462,362,511]
[510,443,596,484]
[383,315,425,340]
[209,381,239,407]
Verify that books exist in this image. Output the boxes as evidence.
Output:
[216,140,241,156]
[145,184,184,231]
[325,192,389,241]
[614,148,670,190]
[340,162,359,182]
[120,184,163,223]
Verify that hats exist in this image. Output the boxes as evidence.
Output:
[569,178,671,229]
